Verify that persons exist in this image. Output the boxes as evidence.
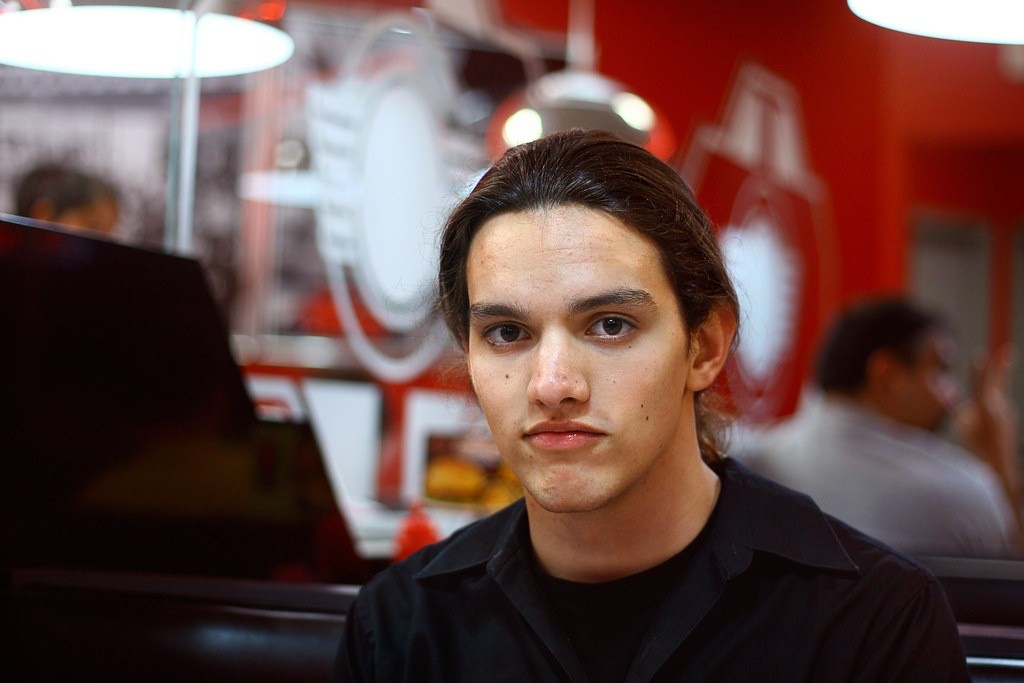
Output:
[329,127,972,683]
[715,293,1024,561]
[9,155,127,232]
